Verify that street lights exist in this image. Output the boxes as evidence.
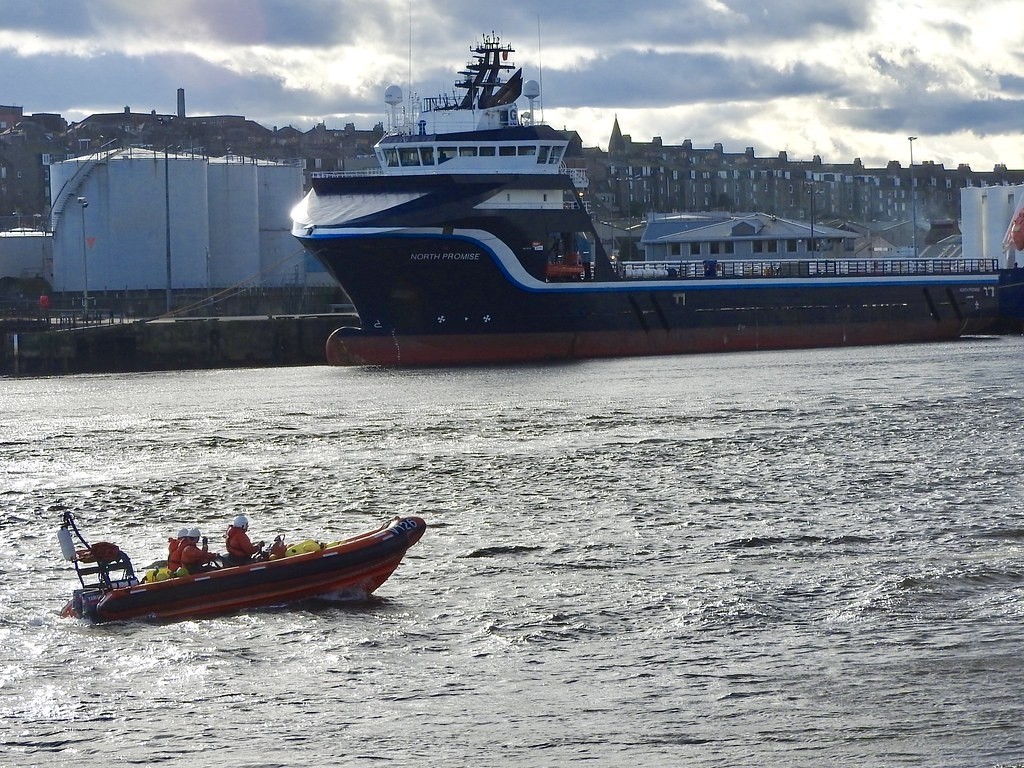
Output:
[77,196,92,317]
[907,136,917,258]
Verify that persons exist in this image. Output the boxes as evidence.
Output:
[225,516,265,568]
[166,527,220,576]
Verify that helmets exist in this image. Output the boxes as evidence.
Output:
[233,516,248,529]
[178,528,189,538]
[187,528,200,538]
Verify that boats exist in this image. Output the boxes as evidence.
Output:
[53,505,429,626]
[286,28,1024,367]
